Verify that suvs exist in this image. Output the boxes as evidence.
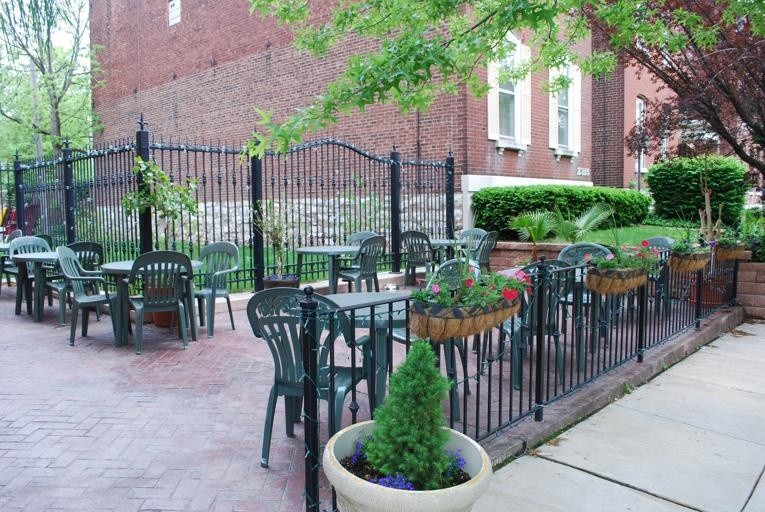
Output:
[1,202,60,240]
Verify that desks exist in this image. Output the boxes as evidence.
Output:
[286,292,414,408]
[495,265,591,373]
[296,245,386,294]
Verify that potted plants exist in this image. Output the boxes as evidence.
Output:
[245,197,299,290]
[320,336,493,512]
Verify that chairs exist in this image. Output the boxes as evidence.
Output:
[1,225,242,352]
[246,286,379,469]
[333,228,499,294]
[639,237,677,318]
[478,259,576,390]
[386,258,481,422]
[557,243,621,355]
